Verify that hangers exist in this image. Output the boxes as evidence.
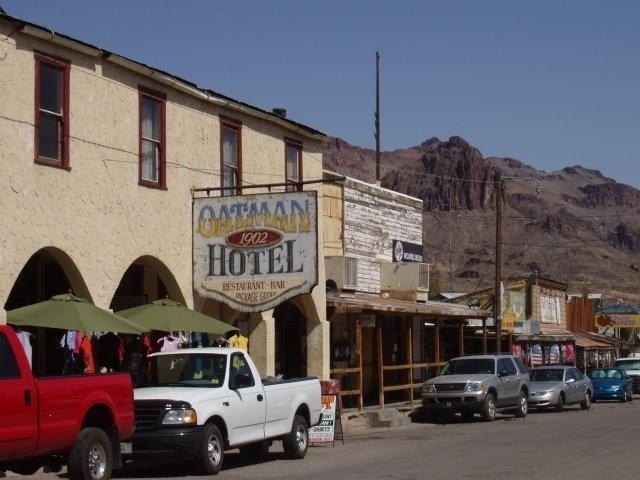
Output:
[13,327,245,342]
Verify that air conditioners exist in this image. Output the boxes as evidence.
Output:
[379,261,430,291]
[323,256,360,290]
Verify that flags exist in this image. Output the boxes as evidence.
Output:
[531,346,544,366]
[550,346,562,364]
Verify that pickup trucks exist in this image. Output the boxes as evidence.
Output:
[0,326,137,480]
[121,347,323,477]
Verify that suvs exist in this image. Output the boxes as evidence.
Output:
[419,354,531,420]
[610,357,639,399]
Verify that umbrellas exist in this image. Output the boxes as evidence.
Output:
[6,289,150,376]
[119,299,242,349]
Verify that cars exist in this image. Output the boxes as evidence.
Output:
[525,365,594,414]
[586,367,634,404]
